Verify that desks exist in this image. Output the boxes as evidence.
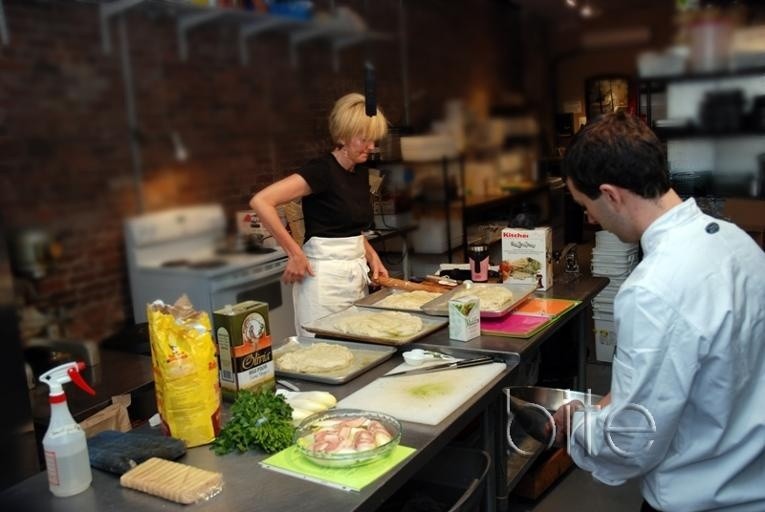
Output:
[0,275,611,512]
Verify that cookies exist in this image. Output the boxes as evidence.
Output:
[121,457,222,505]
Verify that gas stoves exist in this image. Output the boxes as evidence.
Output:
[141,244,287,279]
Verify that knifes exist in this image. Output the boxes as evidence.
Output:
[378,356,494,377]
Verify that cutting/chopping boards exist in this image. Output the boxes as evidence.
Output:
[335,351,507,425]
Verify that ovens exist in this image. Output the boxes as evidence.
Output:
[209,254,297,352]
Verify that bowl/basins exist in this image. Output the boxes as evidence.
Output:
[503,386,605,448]
[402,351,425,366]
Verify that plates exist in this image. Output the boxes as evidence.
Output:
[294,408,403,468]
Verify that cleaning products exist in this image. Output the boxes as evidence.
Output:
[36,361,94,498]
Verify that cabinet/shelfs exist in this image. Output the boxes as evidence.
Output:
[367,106,548,276]
[635,67,765,250]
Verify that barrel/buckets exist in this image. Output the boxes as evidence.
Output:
[589,230,639,362]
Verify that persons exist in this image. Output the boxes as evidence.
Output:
[249,92,389,338]
[544,111,764,512]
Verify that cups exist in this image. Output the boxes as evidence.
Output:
[468,243,490,283]
